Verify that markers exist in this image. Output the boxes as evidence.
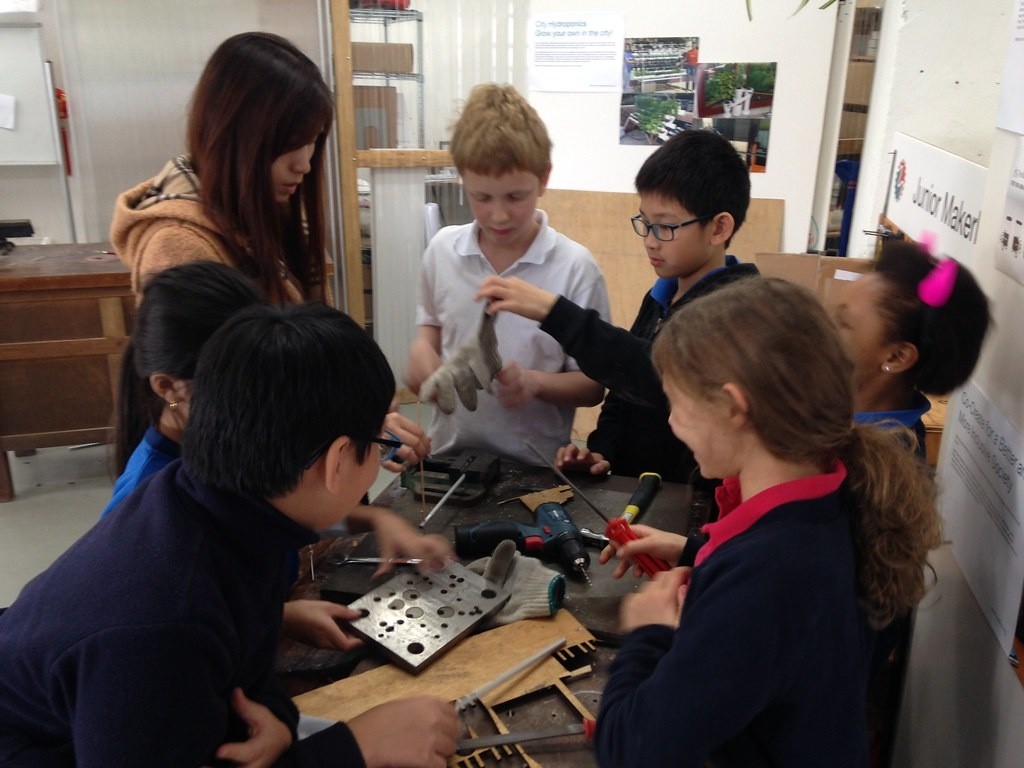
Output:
[54,88,72,177]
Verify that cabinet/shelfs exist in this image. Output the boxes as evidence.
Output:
[349,8,426,151]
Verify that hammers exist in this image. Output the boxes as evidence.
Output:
[576,470,664,553]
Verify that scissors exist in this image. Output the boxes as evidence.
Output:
[323,547,424,566]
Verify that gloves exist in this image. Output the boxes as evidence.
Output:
[465,539,566,625]
[418,303,502,415]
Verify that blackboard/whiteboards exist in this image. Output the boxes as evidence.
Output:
[0,23,66,166]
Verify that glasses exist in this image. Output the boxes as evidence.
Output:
[631,215,699,241]
[303,429,404,470]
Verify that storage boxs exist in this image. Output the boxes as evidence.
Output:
[351,43,414,75]
[844,55,876,105]
[836,111,867,155]
[353,84,397,149]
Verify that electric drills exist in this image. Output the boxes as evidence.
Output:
[453,500,593,587]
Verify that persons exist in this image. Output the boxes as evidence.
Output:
[473,126,764,485]
[623,43,634,91]
[684,42,698,91]
[831,233,992,465]
[0,297,465,768]
[399,78,612,468]
[98,258,458,651]
[108,31,432,506]
[589,271,945,768]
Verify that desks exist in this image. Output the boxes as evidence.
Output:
[274,457,693,768]
[1,242,137,501]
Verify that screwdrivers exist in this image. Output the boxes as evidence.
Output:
[521,437,674,580]
